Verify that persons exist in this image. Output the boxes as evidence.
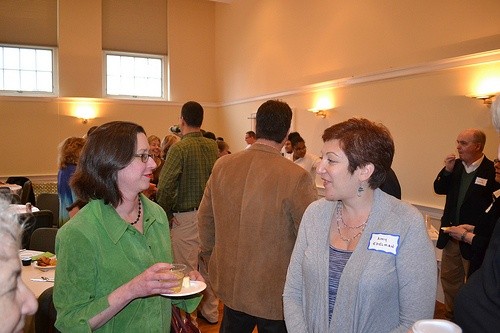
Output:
[433,93,500,333]
[283,118,437,332]
[156,101,220,333]
[284,132,322,184]
[199,100,318,333]
[0,198,39,332]
[53,121,205,333]
[245,131,257,149]
[57,126,231,228]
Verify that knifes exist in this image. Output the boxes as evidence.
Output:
[30,278,55,282]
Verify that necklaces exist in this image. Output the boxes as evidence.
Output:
[340,205,369,228]
[336,200,364,242]
[130,193,141,225]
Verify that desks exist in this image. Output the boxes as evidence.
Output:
[17,249,56,333]
[9,204,40,218]
[0,183,22,197]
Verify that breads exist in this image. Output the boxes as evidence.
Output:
[182,276,191,288]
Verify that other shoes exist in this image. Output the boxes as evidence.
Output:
[197,312,218,324]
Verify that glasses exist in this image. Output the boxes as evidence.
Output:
[136,152,155,162]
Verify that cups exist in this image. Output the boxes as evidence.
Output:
[22,254,32,266]
[412,319,462,333]
[167,264,187,294]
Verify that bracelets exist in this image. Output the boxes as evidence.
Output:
[461,230,469,241]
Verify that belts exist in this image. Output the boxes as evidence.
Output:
[173,208,198,213]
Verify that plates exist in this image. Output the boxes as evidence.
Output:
[161,281,207,297]
[34,257,57,269]
[441,226,456,231]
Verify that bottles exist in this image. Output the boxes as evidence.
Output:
[170,125,181,133]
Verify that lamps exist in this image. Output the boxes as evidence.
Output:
[472,95,496,104]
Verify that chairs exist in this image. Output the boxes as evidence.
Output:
[4,176,61,333]
